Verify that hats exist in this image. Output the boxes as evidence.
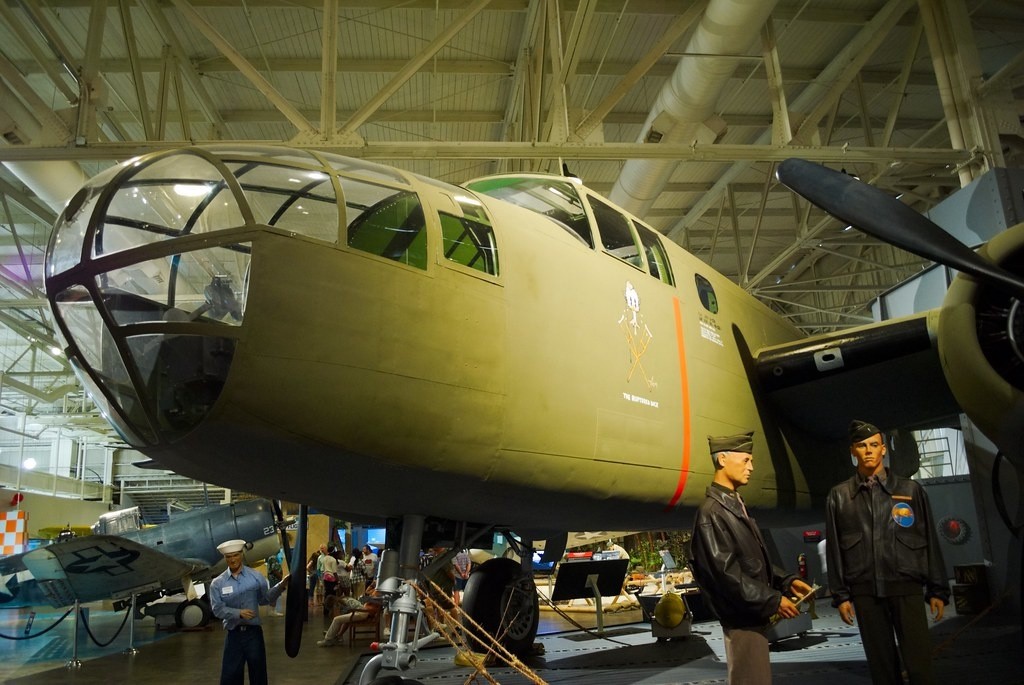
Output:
[709,431,755,454]
[216,539,247,554]
[848,418,883,444]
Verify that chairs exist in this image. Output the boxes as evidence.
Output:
[349,602,382,646]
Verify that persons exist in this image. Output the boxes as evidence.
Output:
[419,548,433,569]
[691,429,816,685]
[431,547,462,641]
[210,539,291,685]
[826,423,951,685]
[451,549,471,606]
[607,542,630,560]
[204,271,242,322]
[307,542,384,647]
[266,549,285,617]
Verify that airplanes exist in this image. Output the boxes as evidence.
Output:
[42,144,1024,685]
[0,499,291,632]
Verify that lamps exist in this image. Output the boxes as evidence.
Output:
[548,162,583,196]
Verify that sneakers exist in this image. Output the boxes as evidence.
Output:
[334,634,344,643]
[317,638,334,646]
